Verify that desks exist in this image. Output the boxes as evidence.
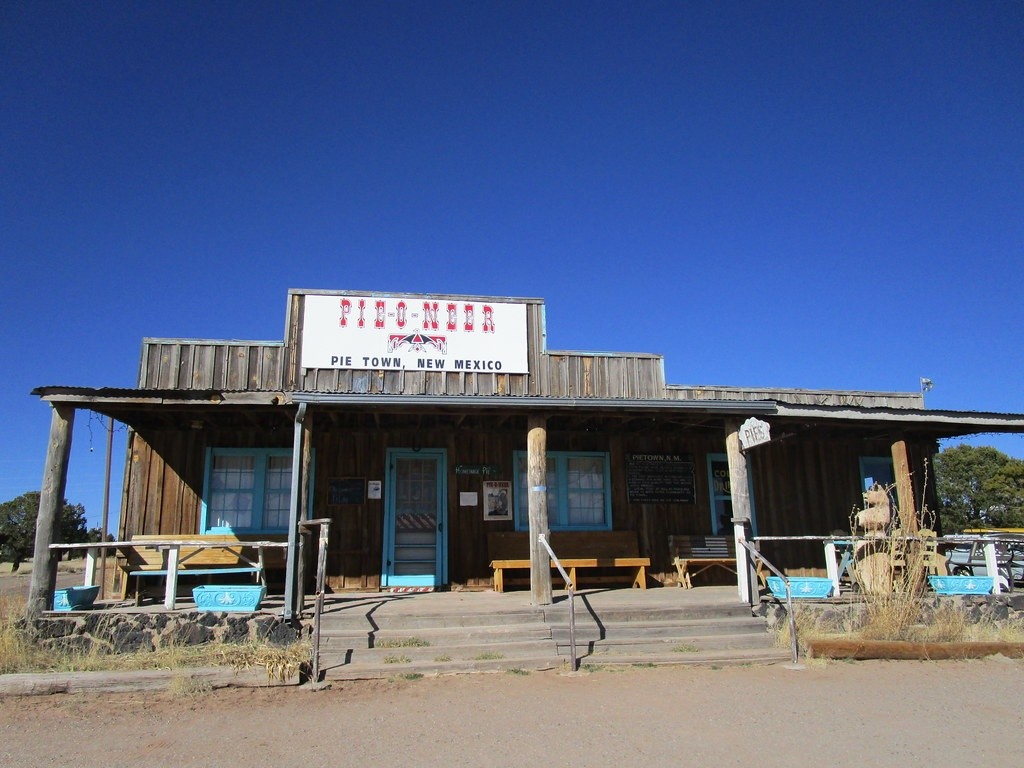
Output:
[960,528,1024,593]
[822,535,1001,595]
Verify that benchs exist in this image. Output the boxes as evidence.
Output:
[488,532,652,593]
[667,534,766,589]
[129,568,263,607]
[114,534,307,599]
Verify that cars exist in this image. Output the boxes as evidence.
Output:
[938,533,1024,581]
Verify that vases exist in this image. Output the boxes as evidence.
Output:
[927,575,994,596]
[192,584,267,613]
[766,576,833,598]
[54,585,101,610]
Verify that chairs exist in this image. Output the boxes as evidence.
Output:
[888,531,939,593]
